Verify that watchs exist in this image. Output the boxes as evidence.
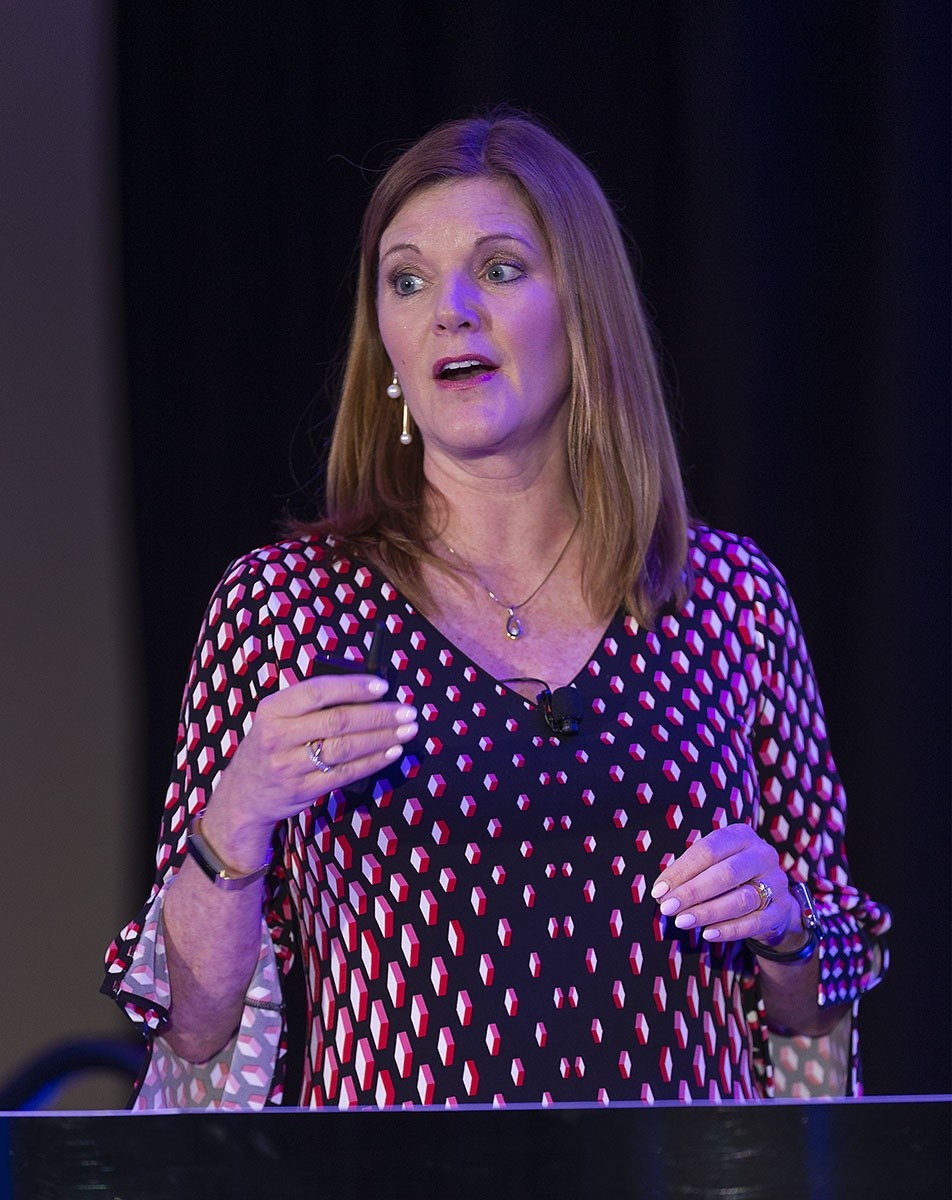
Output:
[745,877,824,965]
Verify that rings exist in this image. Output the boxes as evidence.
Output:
[737,880,773,912]
[303,739,331,773]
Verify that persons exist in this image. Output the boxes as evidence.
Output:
[100,116,891,1110]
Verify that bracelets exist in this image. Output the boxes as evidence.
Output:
[186,807,272,891]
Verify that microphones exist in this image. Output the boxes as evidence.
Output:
[550,686,584,735]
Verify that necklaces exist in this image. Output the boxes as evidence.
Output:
[424,509,583,641]
